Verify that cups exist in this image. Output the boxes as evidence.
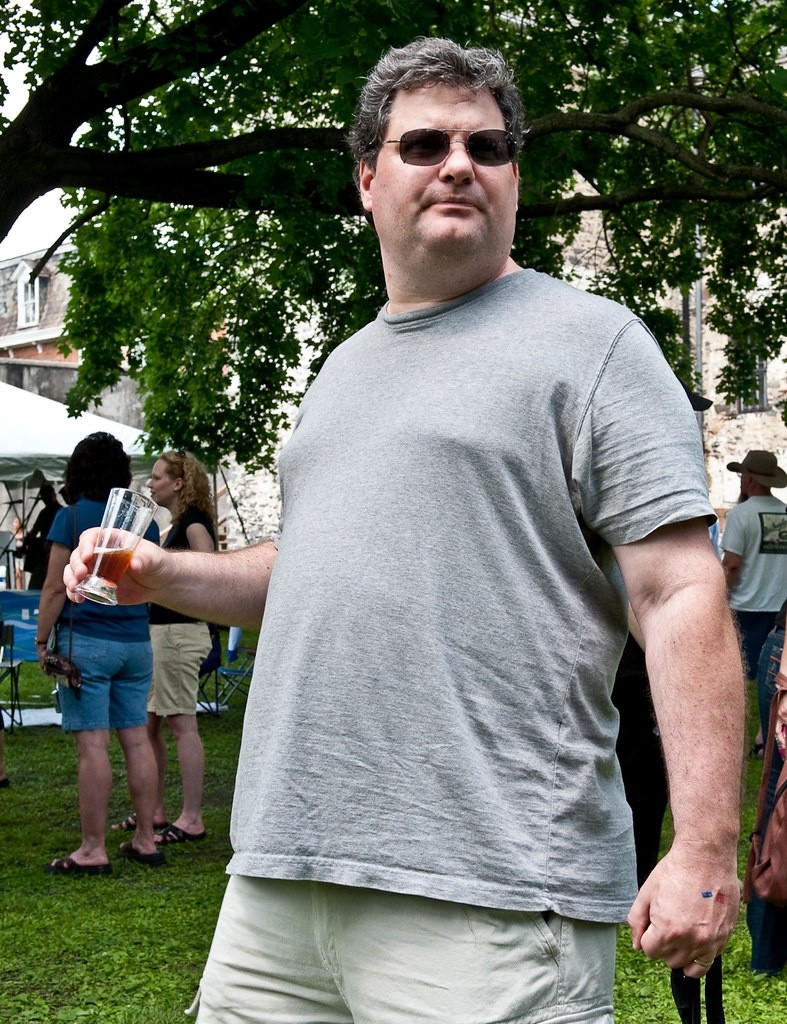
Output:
[71,488,159,607]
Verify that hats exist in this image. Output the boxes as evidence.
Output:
[677,375,713,412]
[39,484,54,495]
[726,449,787,488]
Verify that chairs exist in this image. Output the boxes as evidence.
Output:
[0,624,23,736]
[197,622,257,721]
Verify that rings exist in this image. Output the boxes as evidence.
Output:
[694,959,715,968]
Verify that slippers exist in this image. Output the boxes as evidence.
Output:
[44,854,113,874]
[110,812,170,831]
[116,839,168,866]
[154,824,207,845]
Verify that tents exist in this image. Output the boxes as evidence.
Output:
[0,382,178,483]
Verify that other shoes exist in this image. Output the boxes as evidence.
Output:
[749,743,764,759]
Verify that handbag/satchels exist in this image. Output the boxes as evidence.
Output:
[43,654,81,701]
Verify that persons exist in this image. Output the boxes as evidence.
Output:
[110,450,217,845]
[722,449,787,678]
[62,38,744,1024]
[36,432,160,875]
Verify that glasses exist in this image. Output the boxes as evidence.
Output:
[378,128,519,167]
[736,472,742,477]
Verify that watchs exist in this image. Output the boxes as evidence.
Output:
[34,638,47,646]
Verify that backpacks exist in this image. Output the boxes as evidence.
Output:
[742,671,787,912]
